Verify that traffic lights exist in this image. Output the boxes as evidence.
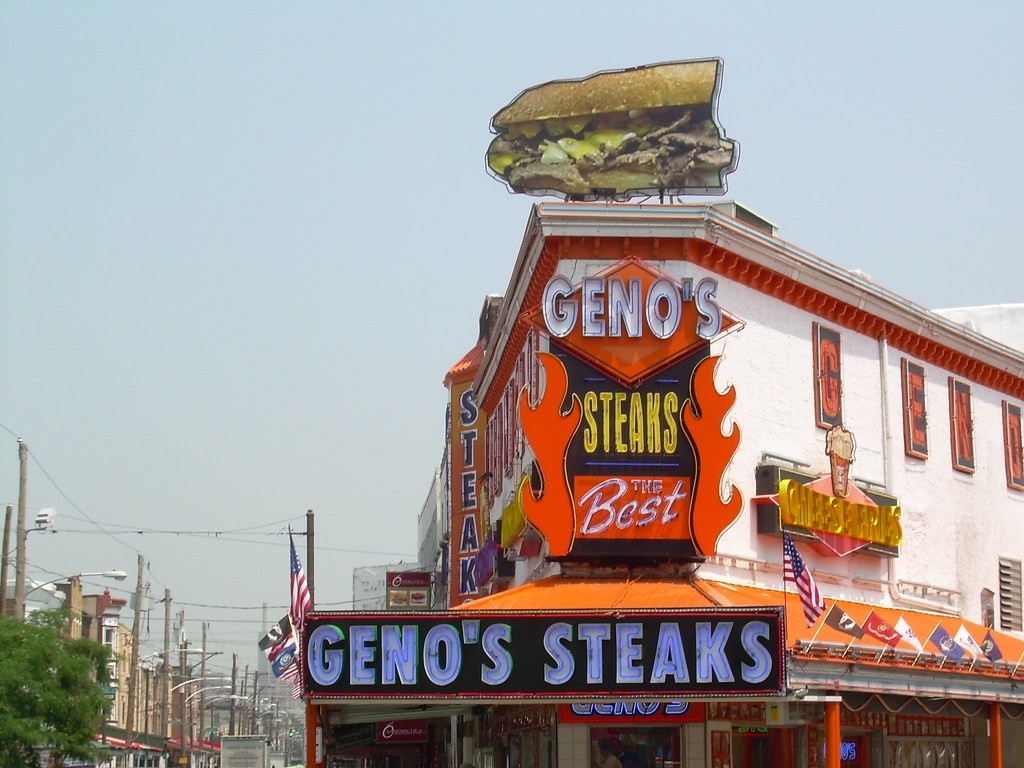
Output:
[289,729,293,737]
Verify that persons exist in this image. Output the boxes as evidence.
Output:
[598,738,639,768]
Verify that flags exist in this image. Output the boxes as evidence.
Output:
[289,542,312,633]
[929,625,965,661]
[784,531,827,628]
[825,605,865,639]
[861,612,902,648]
[257,615,303,700]
[955,626,983,657]
[894,619,923,653]
[980,631,1002,662]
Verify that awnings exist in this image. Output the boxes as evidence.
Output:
[96,727,170,751]
[167,739,221,754]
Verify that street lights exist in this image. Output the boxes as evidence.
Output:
[159,676,277,767]
[125,647,205,748]
[14,570,126,619]
